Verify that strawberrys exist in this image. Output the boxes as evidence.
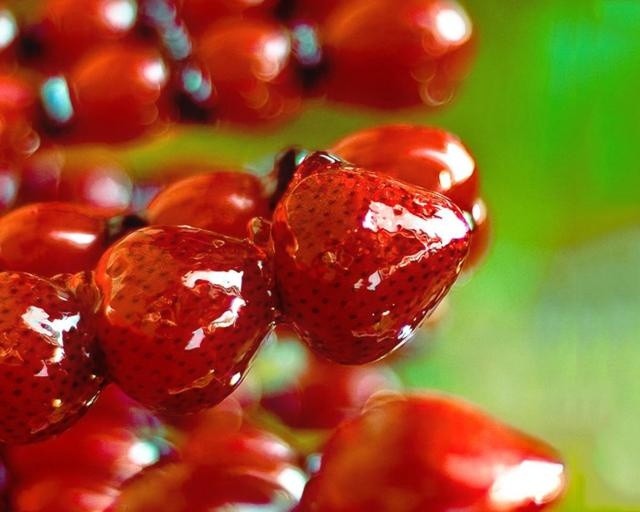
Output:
[0,0,567,512]
